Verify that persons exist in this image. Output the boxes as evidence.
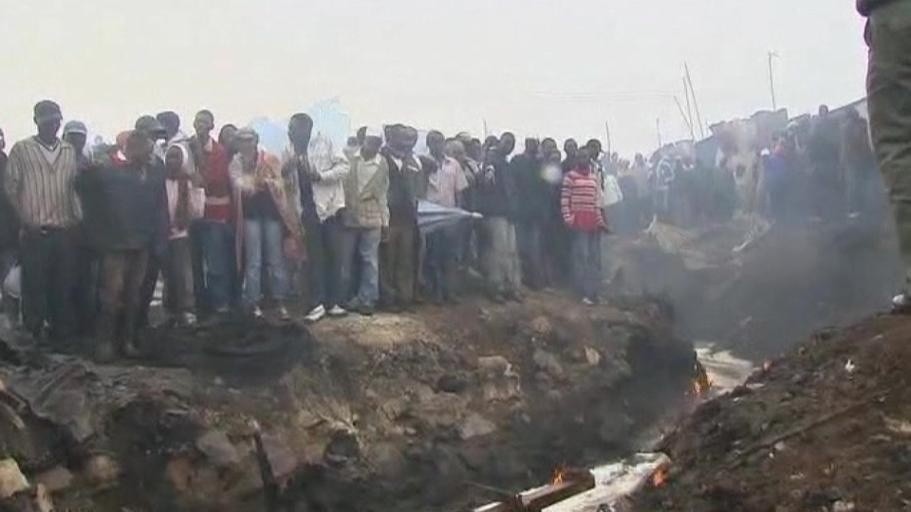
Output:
[853,0,911,312]
[1,100,874,364]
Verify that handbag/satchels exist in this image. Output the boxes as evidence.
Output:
[187,189,206,220]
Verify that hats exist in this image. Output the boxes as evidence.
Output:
[366,119,385,141]
[234,127,257,141]
[63,121,87,134]
[136,117,166,135]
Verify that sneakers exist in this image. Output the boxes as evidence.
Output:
[326,303,347,316]
[302,305,325,322]
